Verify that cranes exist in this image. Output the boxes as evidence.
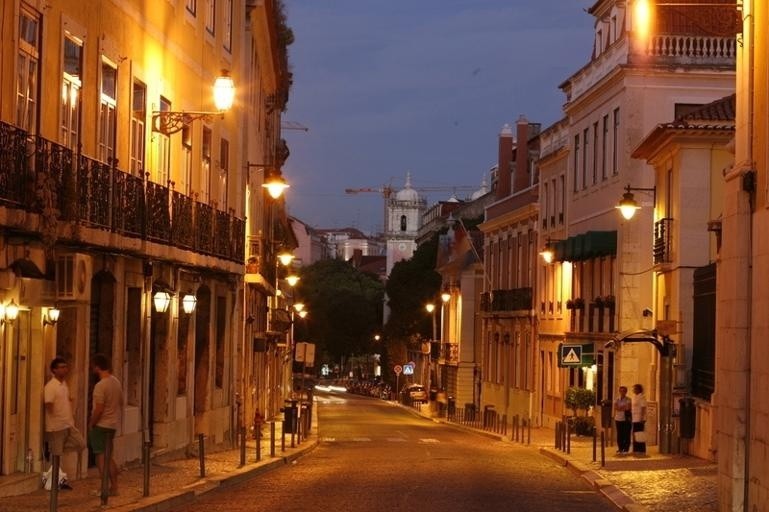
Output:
[345,176,482,233]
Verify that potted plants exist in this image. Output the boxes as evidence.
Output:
[561,386,596,437]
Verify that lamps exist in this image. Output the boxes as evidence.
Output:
[1,256,63,329]
[152,73,237,132]
[614,183,659,223]
[539,237,562,265]
[149,289,199,320]
[246,160,291,200]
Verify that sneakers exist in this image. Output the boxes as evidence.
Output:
[616,450,646,458]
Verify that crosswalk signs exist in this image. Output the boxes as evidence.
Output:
[563,346,582,364]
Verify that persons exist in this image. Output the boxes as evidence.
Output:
[614,384,647,456]
[89,357,122,495]
[44,358,86,490]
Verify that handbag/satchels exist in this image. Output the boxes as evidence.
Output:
[624,411,632,422]
[634,431,648,442]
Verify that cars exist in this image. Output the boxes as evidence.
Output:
[399,383,429,406]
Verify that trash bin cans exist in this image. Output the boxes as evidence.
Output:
[465,403,475,422]
[283,399,298,433]
[448,395,455,419]
[485,405,496,427]
[301,401,312,432]
